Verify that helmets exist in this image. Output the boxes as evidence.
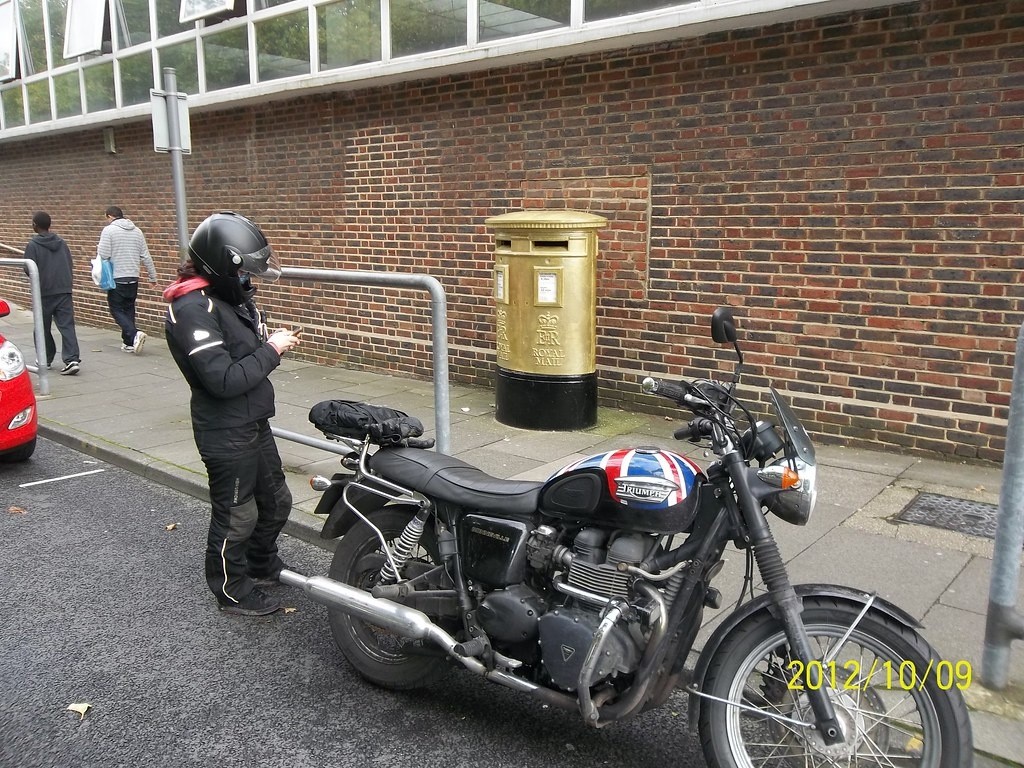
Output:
[187,209,271,305]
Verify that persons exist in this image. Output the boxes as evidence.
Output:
[21,211,82,375]
[163,209,309,615]
[94,205,159,355]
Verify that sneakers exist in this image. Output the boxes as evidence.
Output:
[218,588,280,615]
[60,361,80,376]
[133,330,147,354]
[34,357,51,370]
[120,343,134,354]
[244,564,309,587]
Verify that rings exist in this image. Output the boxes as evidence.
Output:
[288,346,290,350]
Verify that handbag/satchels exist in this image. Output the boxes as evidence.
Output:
[99,257,116,291]
[91,252,102,285]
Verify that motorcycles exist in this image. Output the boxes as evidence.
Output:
[277,303,975,768]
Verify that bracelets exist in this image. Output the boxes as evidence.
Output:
[265,341,278,352]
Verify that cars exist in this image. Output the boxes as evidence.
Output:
[0,300,38,465]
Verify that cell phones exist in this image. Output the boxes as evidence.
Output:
[291,325,303,337]
